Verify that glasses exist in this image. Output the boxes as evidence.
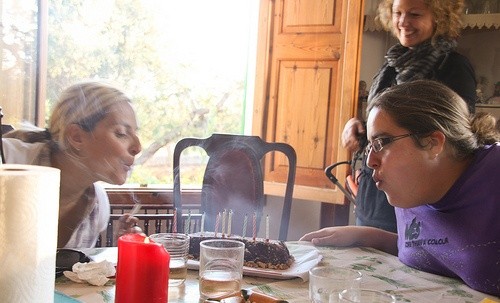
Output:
[365,133,431,157]
[56,248,93,278]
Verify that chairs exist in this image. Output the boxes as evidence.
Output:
[172,133,297,240]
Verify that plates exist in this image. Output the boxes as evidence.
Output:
[188,251,322,280]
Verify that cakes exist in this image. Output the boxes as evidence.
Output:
[163,236,295,270]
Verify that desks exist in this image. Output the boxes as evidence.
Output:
[55,240,500,303]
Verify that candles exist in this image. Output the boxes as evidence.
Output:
[201,210,205,236]
[214,208,232,238]
[253,212,257,240]
[113,233,171,303]
[185,209,191,235]
[174,207,178,232]
[265,214,270,240]
[242,214,248,238]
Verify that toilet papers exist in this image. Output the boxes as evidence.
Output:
[0,163,61,303]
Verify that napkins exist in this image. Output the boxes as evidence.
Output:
[61,260,117,287]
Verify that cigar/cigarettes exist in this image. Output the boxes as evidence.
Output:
[125,203,141,223]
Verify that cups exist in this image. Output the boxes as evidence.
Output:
[338,288,396,303]
[150,233,190,287]
[198,238,245,303]
[308,266,362,303]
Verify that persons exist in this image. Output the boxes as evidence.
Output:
[297,80,500,297]
[0,82,142,250]
[342,0,477,234]
